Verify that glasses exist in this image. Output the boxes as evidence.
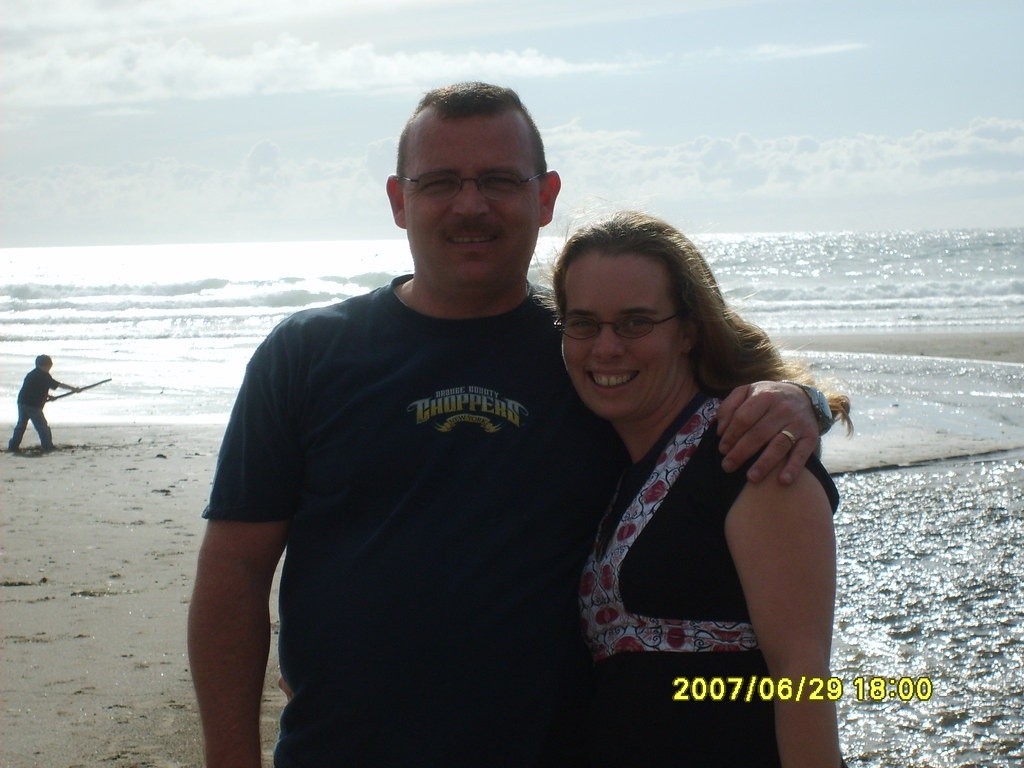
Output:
[398,170,546,202]
[555,311,687,341]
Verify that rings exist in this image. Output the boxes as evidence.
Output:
[780,431,796,444]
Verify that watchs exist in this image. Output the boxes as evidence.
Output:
[782,380,833,436]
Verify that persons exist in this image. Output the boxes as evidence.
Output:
[188,82,853,768]
[8,354,82,453]
